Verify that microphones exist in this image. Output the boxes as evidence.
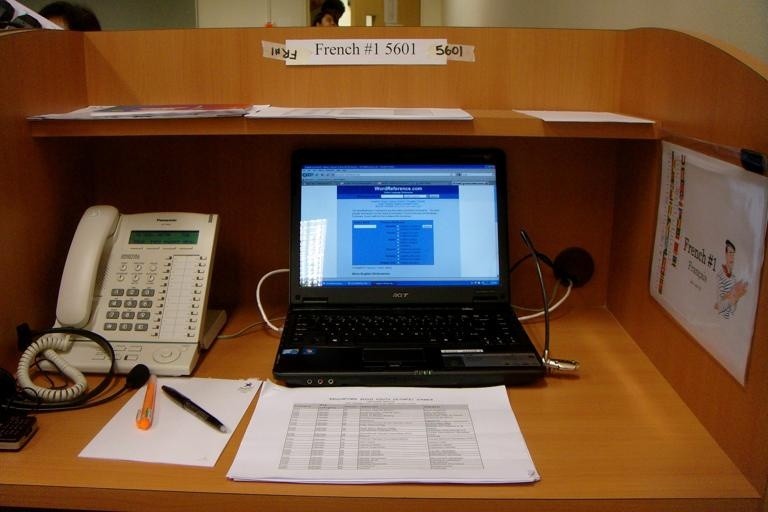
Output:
[4,363,150,412]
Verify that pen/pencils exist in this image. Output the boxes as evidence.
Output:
[203,420,211,422]
[135,373,159,431]
[162,386,228,434]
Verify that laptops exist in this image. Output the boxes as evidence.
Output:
[272,148,547,388]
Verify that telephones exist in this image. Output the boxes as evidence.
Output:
[17,204,220,404]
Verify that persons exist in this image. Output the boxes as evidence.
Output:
[313,12,338,27]
[713,239,748,320]
[34,1,101,32]
[322,0,345,19]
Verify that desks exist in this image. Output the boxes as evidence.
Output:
[0,26,768,512]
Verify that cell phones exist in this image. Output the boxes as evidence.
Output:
[0,415,36,451]
